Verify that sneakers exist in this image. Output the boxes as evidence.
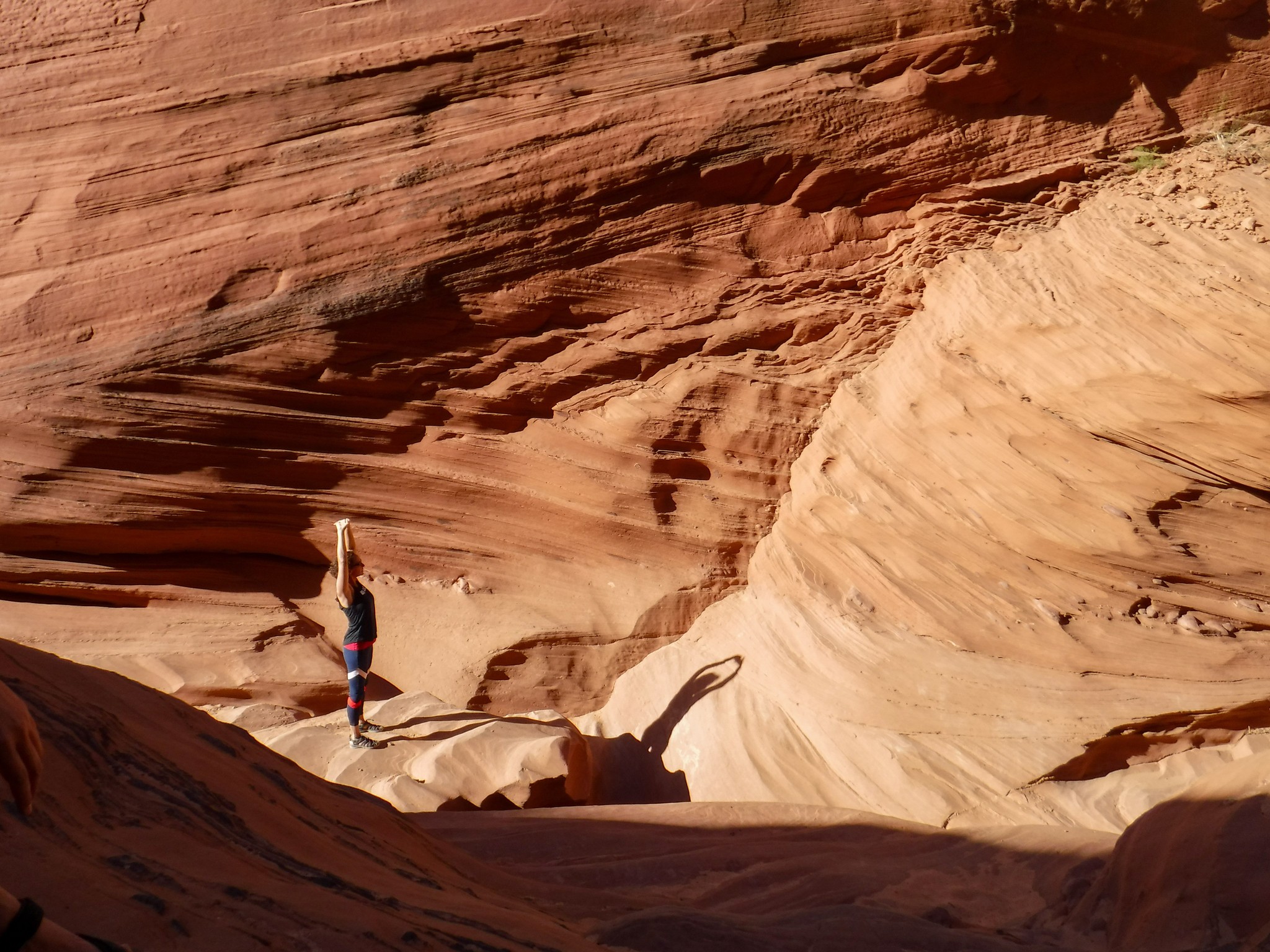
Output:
[349,734,379,749]
[358,719,384,733]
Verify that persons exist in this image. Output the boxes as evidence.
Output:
[333,518,384,749]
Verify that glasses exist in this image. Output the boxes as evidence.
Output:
[349,559,362,568]
